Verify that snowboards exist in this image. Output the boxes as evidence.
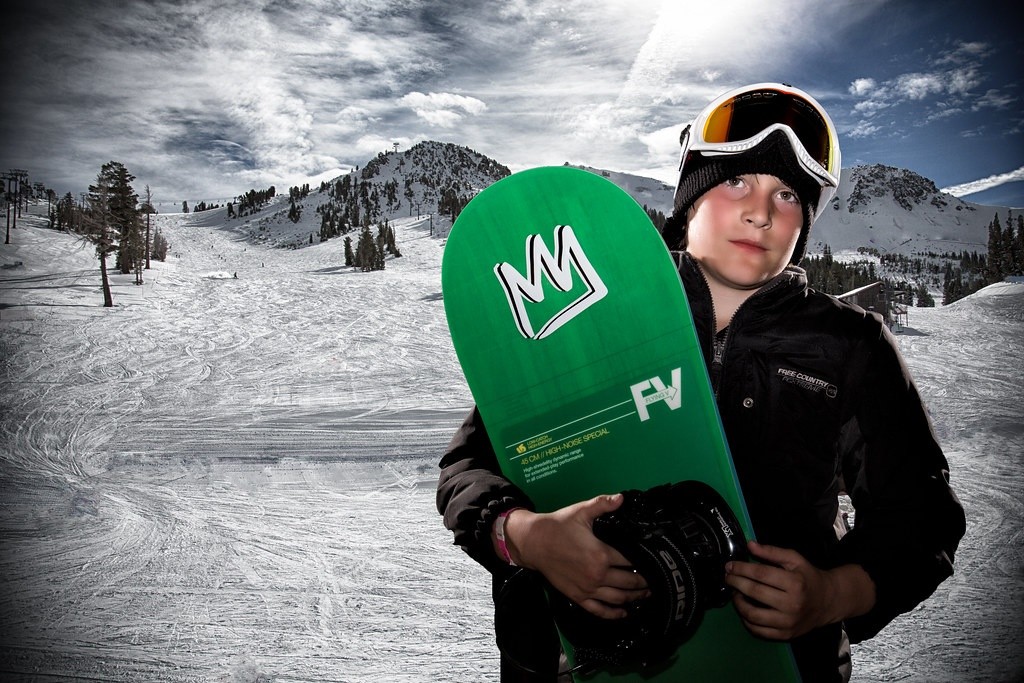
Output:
[441,166,797,683]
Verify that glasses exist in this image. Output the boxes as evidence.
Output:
[688,84,842,189]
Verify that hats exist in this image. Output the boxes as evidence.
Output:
[671,125,815,264]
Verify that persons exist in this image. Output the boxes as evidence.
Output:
[234,272,237,278]
[435,82,965,683]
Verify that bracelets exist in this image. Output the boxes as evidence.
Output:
[495,508,525,566]
